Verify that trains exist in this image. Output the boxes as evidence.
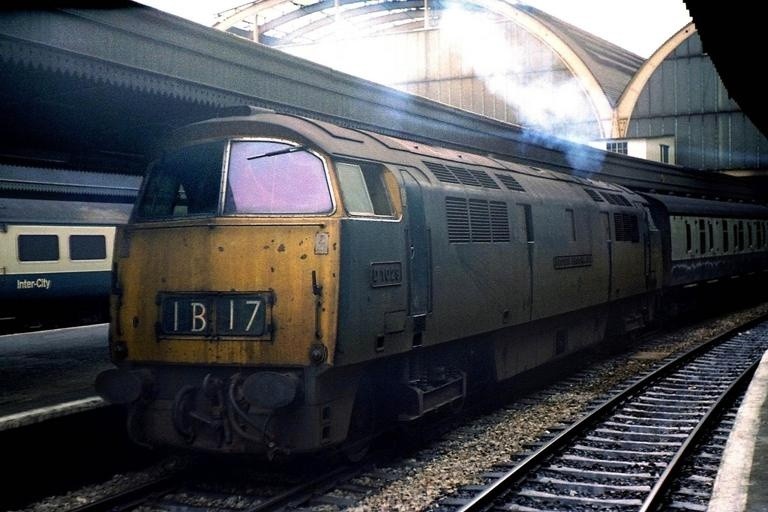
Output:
[0,197,137,326]
[97,106,768,490]
